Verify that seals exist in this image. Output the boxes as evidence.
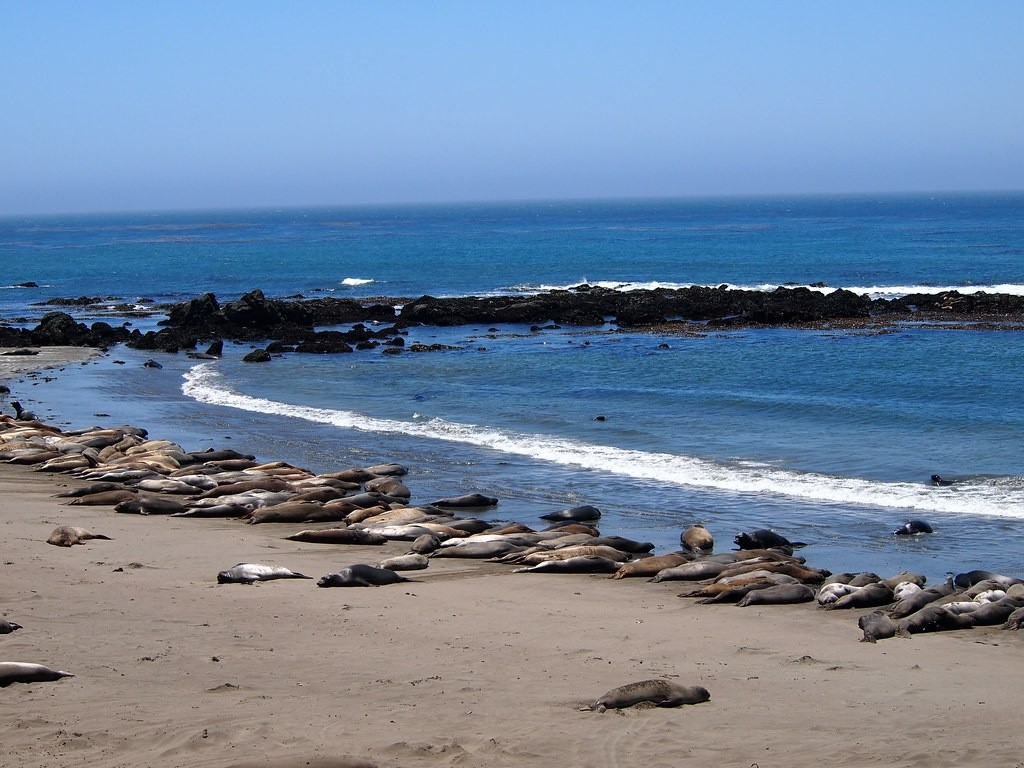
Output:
[0,411,1024,643]
[580,678,710,712]
[0,661,75,694]
